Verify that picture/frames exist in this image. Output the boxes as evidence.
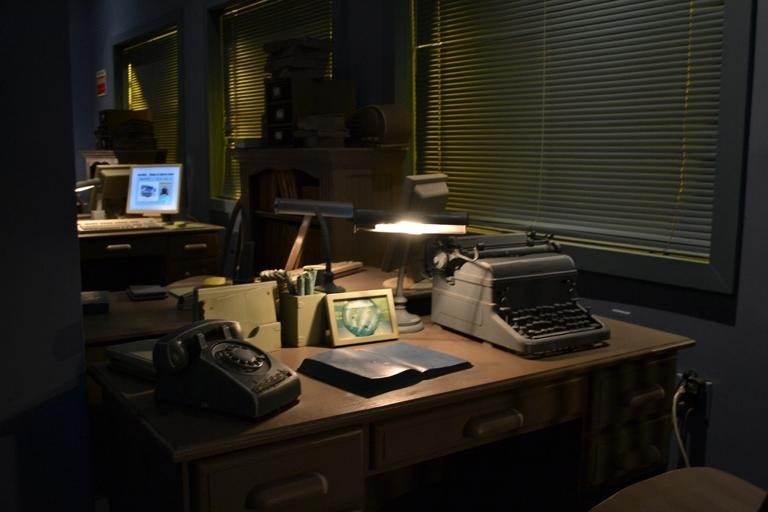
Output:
[324,288,400,347]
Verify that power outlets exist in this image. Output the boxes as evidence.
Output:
[675,372,712,419]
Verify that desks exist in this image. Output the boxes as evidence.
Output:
[80,291,195,360]
[77,217,226,284]
[85,312,696,512]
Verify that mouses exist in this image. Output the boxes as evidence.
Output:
[174,220,186,228]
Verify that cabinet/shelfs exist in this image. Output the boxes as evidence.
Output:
[232,144,409,270]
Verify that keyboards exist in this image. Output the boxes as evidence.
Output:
[287,261,364,290]
[76,218,165,234]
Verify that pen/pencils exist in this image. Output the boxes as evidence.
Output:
[274,269,313,296]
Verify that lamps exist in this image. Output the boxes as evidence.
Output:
[353,208,469,335]
[273,198,353,294]
[73,177,106,219]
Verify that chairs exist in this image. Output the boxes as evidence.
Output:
[164,200,249,288]
[589,465,768,512]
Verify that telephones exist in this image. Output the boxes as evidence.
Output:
[152,319,301,418]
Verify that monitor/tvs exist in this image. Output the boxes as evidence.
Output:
[382,173,450,296]
[125,163,183,225]
[87,164,143,219]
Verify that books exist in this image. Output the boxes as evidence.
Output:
[296,341,469,396]
[129,284,167,297]
[105,338,159,382]
[126,289,168,302]
